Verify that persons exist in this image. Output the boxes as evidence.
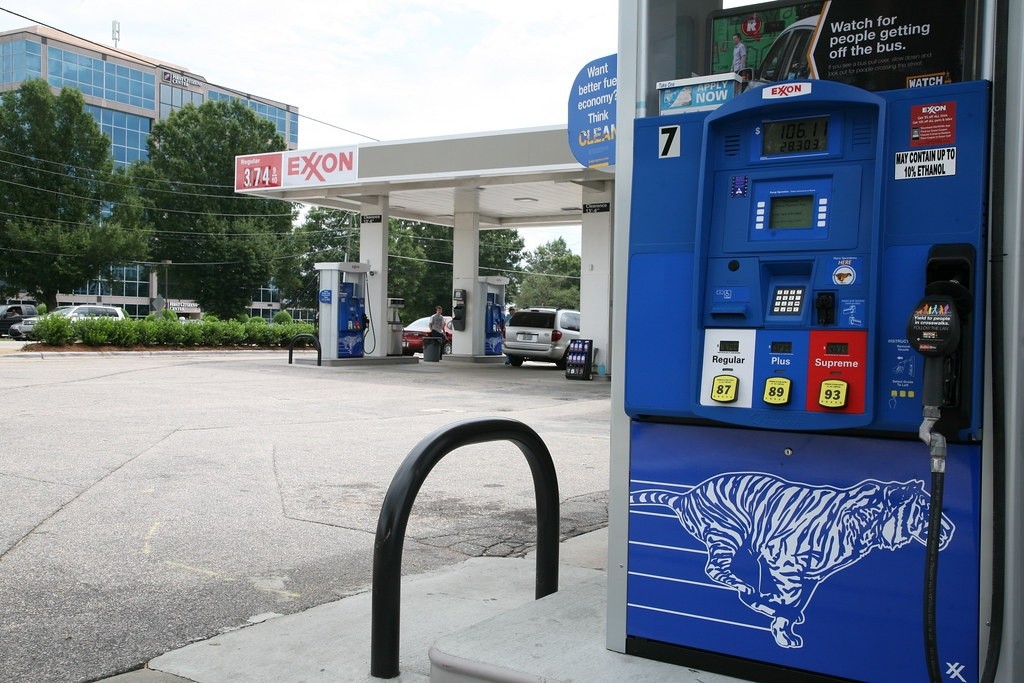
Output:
[504,307,515,365]
[730,34,746,74]
[10,309,19,317]
[429,306,446,360]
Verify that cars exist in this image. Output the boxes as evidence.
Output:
[401,314,453,356]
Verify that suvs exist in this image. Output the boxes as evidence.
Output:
[21,305,125,341]
[502,305,580,370]
[0,305,39,337]
[8,306,78,341]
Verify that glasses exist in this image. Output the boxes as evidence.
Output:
[510,309,514,311]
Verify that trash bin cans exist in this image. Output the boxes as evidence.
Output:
[422,337,442,362]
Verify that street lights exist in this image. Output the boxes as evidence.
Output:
[162,260,172,320]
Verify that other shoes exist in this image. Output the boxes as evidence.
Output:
[505,362,510,365]
